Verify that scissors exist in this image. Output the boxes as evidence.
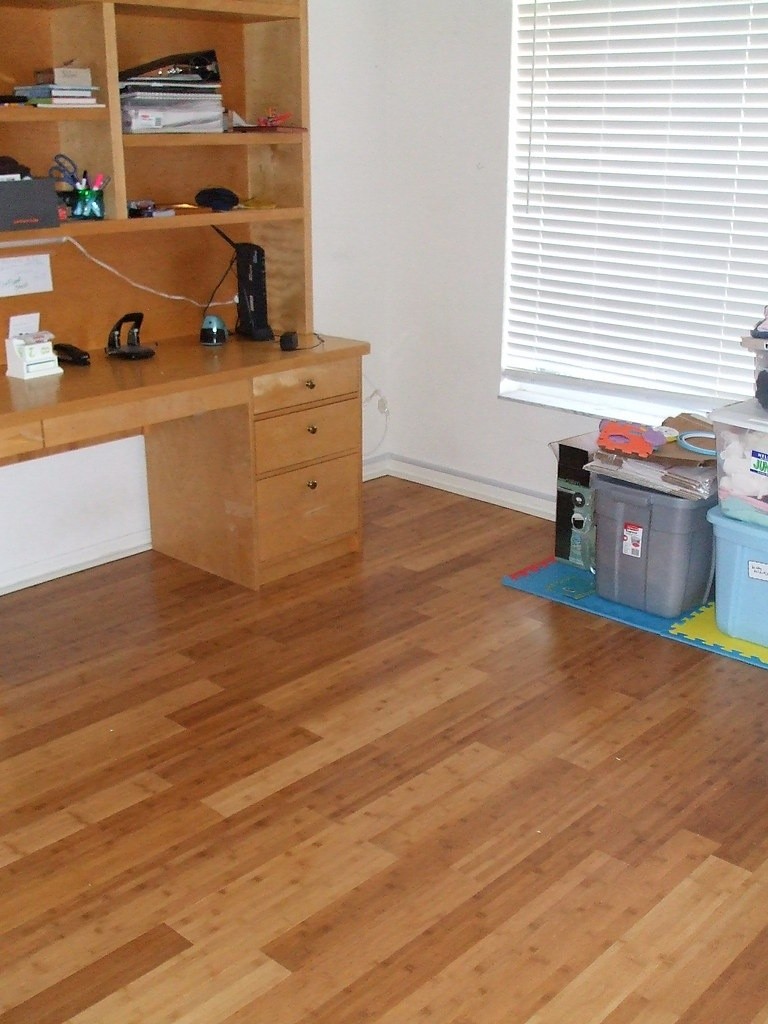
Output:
[48,155,80,187]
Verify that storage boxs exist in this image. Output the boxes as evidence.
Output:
[0,176,60,233]
[549,430,599,570]
[710,397,768,529]
[588,475,718,620]
[122,98,223,133]
[705,504,768,647]
[35,67,93,87]
[4,338,64,381]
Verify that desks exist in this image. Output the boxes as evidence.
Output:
[0,329,372,593]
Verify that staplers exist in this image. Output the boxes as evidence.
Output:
[54,343,91,366]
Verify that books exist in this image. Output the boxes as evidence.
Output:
[118,82,227,134]
[12,84,106,108]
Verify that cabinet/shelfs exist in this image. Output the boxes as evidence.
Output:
[0,0,315,367]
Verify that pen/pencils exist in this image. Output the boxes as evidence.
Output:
[70,166,112,219]
